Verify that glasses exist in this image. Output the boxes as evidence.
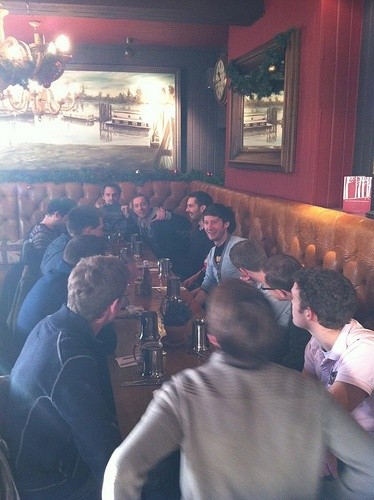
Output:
[260,283,278,290]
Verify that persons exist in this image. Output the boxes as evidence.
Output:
[0,183,374,500]
[100,277,374,500]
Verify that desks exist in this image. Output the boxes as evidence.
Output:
[105,242,212,445]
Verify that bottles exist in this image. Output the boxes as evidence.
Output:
[141,260,152,296]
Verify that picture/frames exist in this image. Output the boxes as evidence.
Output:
[0,65,180,182]
[230,30,301,172]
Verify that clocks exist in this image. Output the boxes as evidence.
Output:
[214,55,230,104]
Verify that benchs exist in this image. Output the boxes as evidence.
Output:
[0,182,374,306]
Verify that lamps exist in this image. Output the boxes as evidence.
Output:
[0,88,74,122]
[0,4,71,90]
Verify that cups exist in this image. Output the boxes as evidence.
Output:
[138,311,160,341]
[167,277,181,299]
[133,242,144,257]
[191,319,210,352]
[134,278,141,296]
[130,233,139,248]
[120,246,130,263]
[157,257,171,277]
[133,341,166,378]
